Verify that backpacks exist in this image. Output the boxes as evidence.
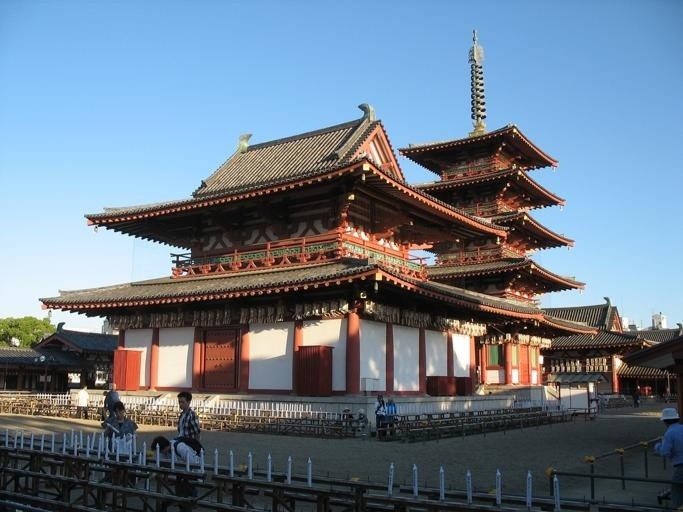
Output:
[173,436,205,457]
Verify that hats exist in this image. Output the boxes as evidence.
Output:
[358,408,365,414]
[660,408,680,421]
[342,406,350,412]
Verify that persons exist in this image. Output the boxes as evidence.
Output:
[384,397,396,435]
[342,407,353,426]
[375,395,386,428]
[102,383,204,512]
[632,391,639,408]
[74,386,89,419]
[654,408,683,508]
[356,409,368,432]
